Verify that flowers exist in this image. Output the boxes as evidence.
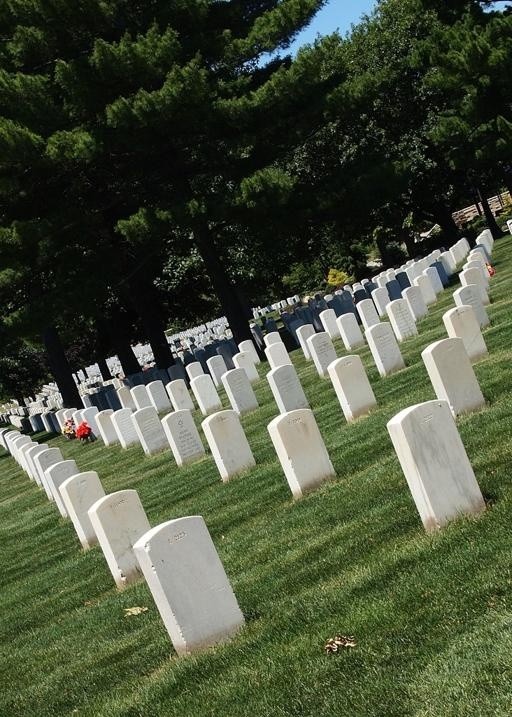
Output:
[62,417,76,439]
[485,263,495,276]
[75,423,97,444]
